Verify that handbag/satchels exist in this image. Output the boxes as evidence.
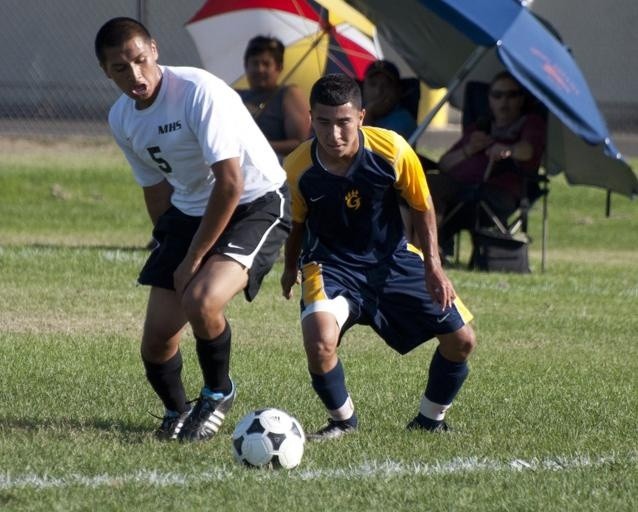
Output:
[473,229,532,274]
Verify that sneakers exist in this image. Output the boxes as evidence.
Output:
[178,378,237,443]
[406,416,457,433]
[305,417,359,441]
[147,406,194,441]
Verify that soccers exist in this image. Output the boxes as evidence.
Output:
[230,407,307,473]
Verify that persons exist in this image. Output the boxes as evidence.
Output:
[92,15,295,441]
[226,34,549,264]
[276,71,476,443]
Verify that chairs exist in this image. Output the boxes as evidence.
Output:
[353,77,551,271]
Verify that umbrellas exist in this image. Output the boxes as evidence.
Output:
[362,1,637,200]
[185,0,385,126]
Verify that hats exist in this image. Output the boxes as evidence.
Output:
[367,61,400,81]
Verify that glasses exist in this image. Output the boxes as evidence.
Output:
[490,90,520,98]
